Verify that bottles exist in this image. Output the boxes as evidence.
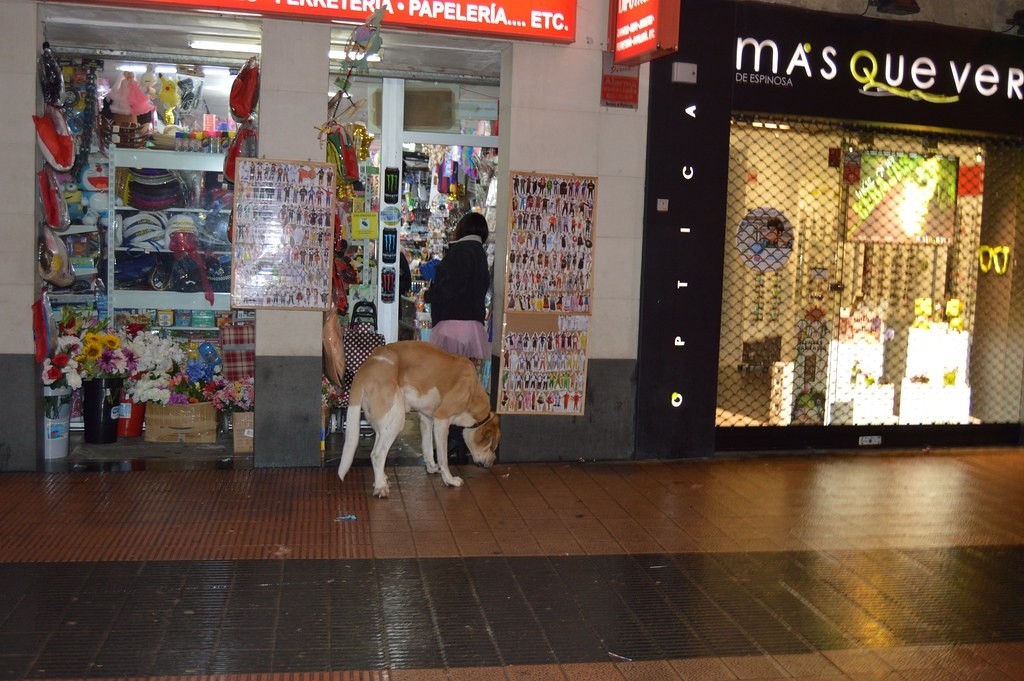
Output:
[199,341,220,366]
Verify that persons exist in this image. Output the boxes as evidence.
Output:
[422,212,492,465]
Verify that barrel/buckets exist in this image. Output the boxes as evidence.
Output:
[117,398,145,436]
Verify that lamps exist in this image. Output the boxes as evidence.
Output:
[863,0,922,16]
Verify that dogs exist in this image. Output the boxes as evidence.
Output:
[336,339,503,500]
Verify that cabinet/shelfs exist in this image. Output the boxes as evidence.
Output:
[399,163,432,343]
[107,144,239,362]
[35,149,103,430]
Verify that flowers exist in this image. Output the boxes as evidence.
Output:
[42,302,256,414]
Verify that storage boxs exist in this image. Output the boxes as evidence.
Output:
[157,309,173,328]
[234,413,255,453]
[174,308,191,327]
[144,401,218,444]
[192,309,216,328]
[216,310,233,329]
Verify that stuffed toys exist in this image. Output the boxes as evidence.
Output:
[55,173,85,224]
[78,163,124,226]
[104,63,182,125]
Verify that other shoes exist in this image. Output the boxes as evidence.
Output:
[417,439,469,465]
